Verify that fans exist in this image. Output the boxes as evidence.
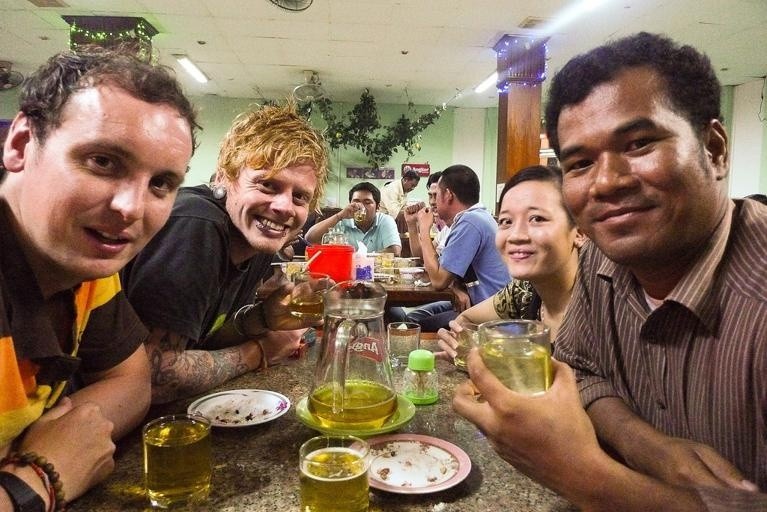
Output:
[292,70,327,102]
[269,0,313,11]
[0,62,24,92]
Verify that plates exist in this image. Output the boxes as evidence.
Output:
[188,388,290,428]
[295,381,416,438]
[350,433,471,496]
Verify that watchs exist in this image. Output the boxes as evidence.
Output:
[0,469,45,512]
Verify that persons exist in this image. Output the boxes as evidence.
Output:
[450,14,767,512]
[120,96,335,408]
[402,164,511,333]
[384,171,451,330]
[378,171,419,231]
[432,157,588,374]
[304,182,403,258]
[0,45,204,512]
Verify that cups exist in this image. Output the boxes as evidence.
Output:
[298,435,372,512]
[142,415,212,512]
[478,320,554,396]
[453,323,480,371]
[353,209,367,222]
[387,323,420,367]
[290,273,329,321]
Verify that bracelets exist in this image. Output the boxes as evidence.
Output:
[254,338,269,369]
[233,303,254,339]
[2,448,69,512]
[337,212,343,221]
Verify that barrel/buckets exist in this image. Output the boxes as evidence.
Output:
[306,244,355,289]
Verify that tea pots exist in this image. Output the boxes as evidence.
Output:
[307,279,398,430]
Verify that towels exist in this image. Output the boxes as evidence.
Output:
[351,238,375,282]
